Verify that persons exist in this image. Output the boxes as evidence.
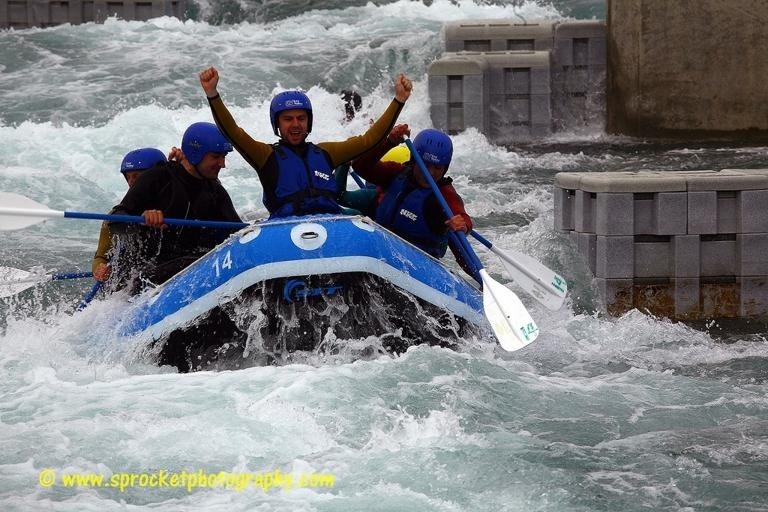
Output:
[350,123,473,266]
[91,144,187,284]
[199,64,413,220]
[339,144,413,214]
[110,122,245,299]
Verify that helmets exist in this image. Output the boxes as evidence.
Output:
[270,91,312,135]
[121,148,167,172]
[181,122,233,165]
[380,129,453,175]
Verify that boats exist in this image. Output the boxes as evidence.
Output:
[110,212,500,373]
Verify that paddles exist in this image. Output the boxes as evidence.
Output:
[0,263,95,298]
[398,123,540,354]
[465,229,569,315]
[0,190,250,230]
